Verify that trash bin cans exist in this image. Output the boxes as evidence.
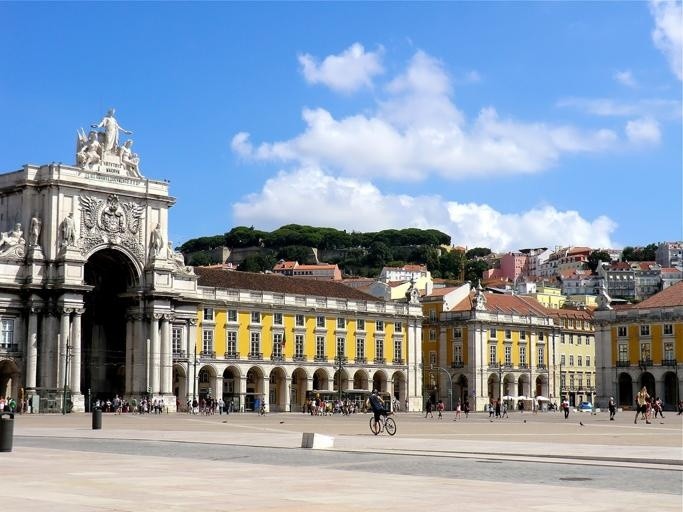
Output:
[92,406,102,429]
[1,412,14,452]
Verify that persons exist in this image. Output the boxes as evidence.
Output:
[302,396,408,416]
[596,387,682,424]
[424,398,570,420]
[176,396,265,416]
[90,394,165,415]
[7,397,32,414]
[366,389,389,434]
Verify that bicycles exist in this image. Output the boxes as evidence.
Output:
[366,405,396,435]
